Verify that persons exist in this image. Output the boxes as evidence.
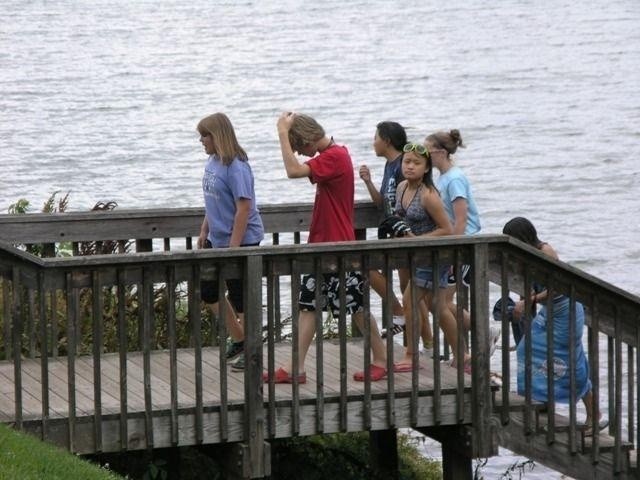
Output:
[361,120,412,341]
[257,109,394,383]
[421,127,501,368]
[194,111,267,373]
[502,215,610,437]
[391,142,475,377]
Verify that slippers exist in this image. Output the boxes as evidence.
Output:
[263,366,306,384]
[354,362,424,382]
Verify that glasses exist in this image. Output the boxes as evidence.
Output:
[403,144,444,158]
[198,134,213,138]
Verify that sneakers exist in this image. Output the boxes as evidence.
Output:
[490,321,500,358]
[583,415,609,437]
[382,323,405,338]
[226,340,246,372]
